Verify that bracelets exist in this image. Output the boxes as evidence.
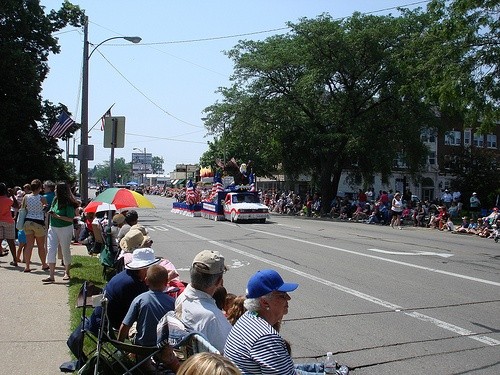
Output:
[61,216,64,220]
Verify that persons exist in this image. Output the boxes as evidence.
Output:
[0,179,500,375]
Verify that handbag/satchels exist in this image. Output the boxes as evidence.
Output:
[16,196,27,230]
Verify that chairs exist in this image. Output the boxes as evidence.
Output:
[100,228,118,279]
[95,298,170,375]
[89,224,105,252]
[167,311,222,358]
[66,281,139,370]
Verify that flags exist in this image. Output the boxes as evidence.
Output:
[48,110,75,140]
[101,109,112,131]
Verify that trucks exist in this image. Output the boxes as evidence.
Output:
[199,188,271,224]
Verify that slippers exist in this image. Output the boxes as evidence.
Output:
[41,277,55,282]
[24,268,31,272]
[63,276,70,280]
[42,266,49,270]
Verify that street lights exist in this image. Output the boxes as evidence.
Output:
[133,147,146,194]
[79,15,142,206]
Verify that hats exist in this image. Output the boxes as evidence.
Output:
[191,249,231,274]
[112,214,126,225]
[16,190,24,196]
[245,269,300,298]
[124,247,162,270]
[124,223,149,237]
[119,229,151,252]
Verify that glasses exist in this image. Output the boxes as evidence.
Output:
[274,291,286,297]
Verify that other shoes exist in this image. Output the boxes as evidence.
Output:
[10,261,17,266]
[0,251,8,257]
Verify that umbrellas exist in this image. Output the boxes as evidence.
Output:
[93,187,157,214]
[84,201,127,213]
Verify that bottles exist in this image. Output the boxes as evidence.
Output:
[324,352,337,375]
